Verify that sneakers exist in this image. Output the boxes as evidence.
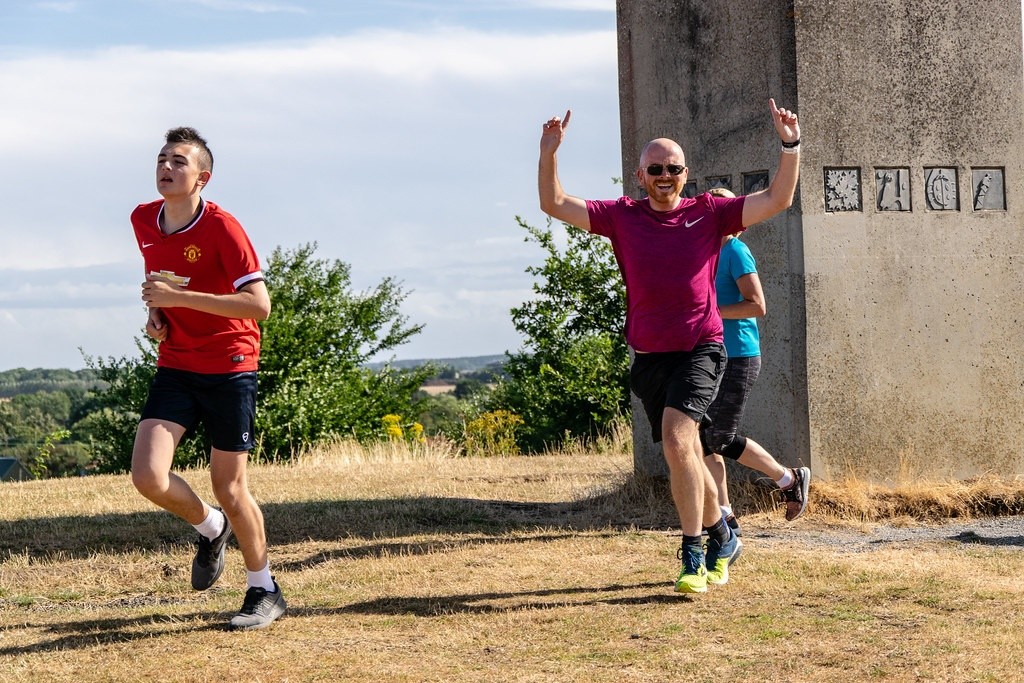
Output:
[779,467,812,521]
[191,507,234,591]
[701,527,744,585]
[674,545,709,593]
[227,576,288,630]
[701,511,742,535]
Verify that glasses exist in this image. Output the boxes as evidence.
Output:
[639,163,686,176]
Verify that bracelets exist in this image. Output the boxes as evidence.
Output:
[780,139,801,154]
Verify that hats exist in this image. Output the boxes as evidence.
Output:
[709,188,736,198]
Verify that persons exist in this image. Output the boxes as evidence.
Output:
[538,99,801,593]
[130,128,287,630]
[700,187,810,536]
